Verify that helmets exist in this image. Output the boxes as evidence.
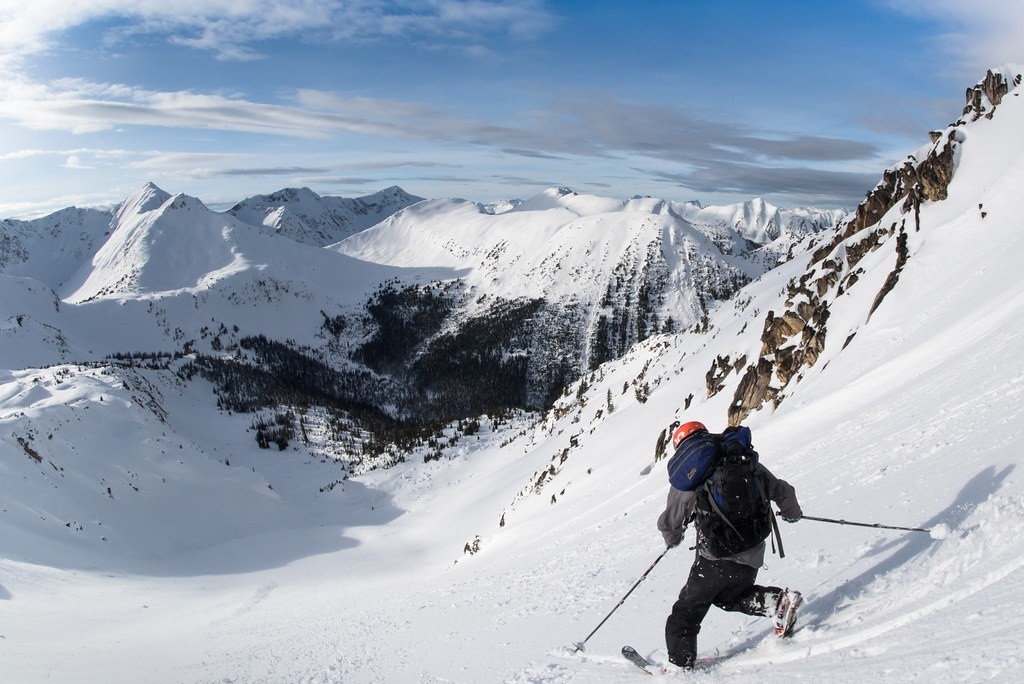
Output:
[673,421,707,451]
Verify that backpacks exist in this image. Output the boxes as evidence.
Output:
[667,426,772,558]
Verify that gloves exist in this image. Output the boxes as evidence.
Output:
[783,511,802,523]
[669,534,685,548]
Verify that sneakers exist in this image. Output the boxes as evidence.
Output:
[775,587,803,639]
[645,661,694,680]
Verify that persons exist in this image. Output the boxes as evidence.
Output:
[643,421,803,681]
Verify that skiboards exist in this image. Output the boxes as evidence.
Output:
[621,589,803,679]
